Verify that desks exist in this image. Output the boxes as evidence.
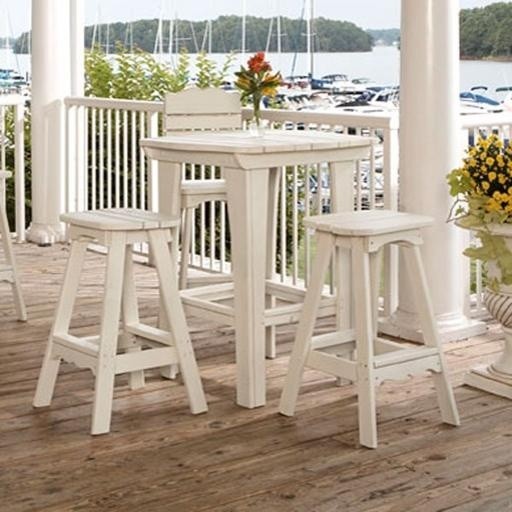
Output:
[137,127,378,412]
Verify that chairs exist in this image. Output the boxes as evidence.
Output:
[151,86,283,358]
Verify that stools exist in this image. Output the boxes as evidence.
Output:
[30,208,212,447]
[276,207,459,451]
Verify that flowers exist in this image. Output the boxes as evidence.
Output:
[230,54,287,128]
[449,133,511,278]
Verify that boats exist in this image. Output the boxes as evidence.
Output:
[300,66,512,204]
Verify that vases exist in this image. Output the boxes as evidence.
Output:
[452,214,511,398]
[251,111,265,136]
